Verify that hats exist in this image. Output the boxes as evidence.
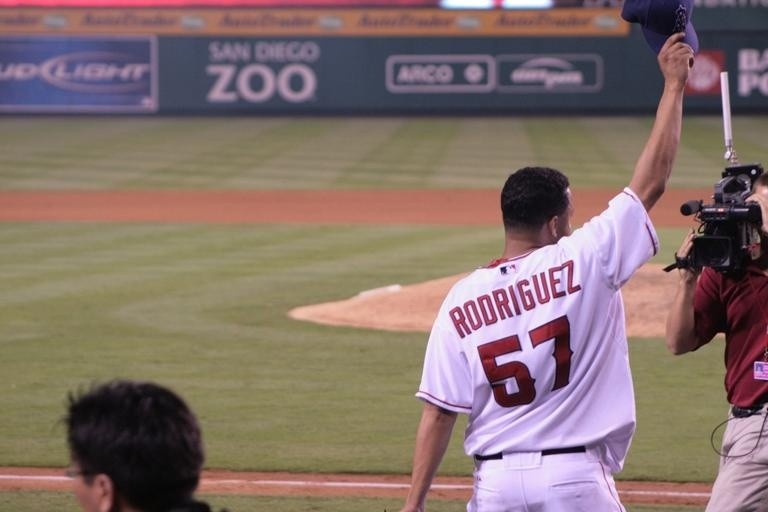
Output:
[621,0,699,56]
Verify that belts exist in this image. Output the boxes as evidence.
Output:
[473,446,588,460]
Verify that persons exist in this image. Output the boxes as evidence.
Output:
[666,173,768,512]
[67,381,224,512]
[399,32,697,512]
